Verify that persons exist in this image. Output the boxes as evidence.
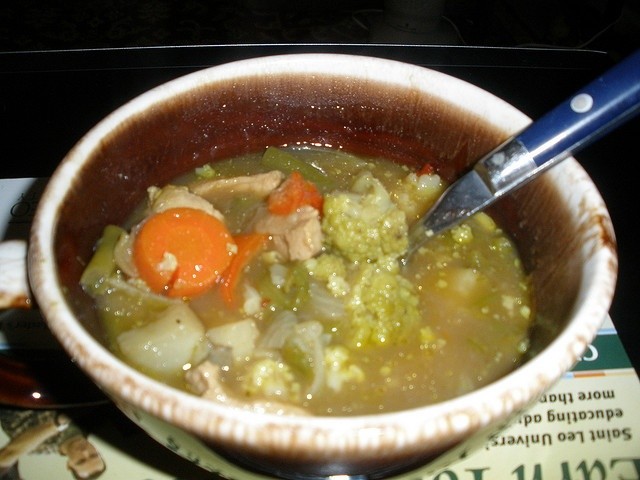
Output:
[0,404,105,476]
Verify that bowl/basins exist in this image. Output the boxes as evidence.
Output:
[26,49,619,479]
